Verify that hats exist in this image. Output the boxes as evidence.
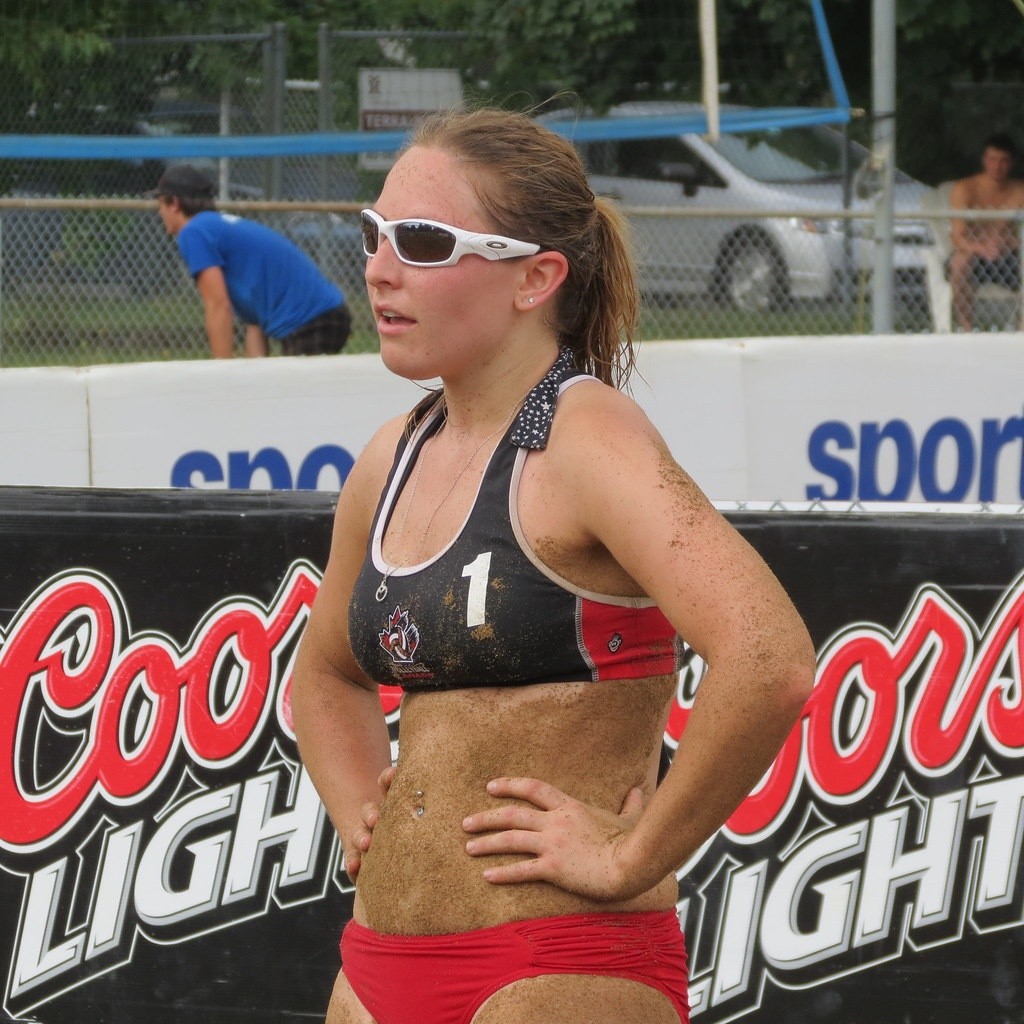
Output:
[143,166,213,198]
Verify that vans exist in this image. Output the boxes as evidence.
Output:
[533,98,950,316]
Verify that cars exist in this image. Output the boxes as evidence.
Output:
[0,100,368,281]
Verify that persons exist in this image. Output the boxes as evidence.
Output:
[943,133,1024,329]
[289,110,817,1023]
[146,166,350,359]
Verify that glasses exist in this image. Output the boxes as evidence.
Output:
[361,208,543,267]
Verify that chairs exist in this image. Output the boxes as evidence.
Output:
[924,179,1024,334]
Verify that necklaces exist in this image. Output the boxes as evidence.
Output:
[376,387,533,602]
